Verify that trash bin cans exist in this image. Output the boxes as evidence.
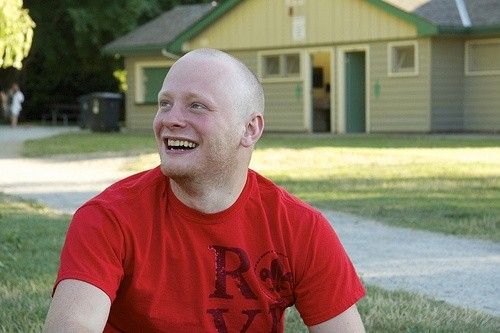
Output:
[77,94,91,129]
[87,92,123,132]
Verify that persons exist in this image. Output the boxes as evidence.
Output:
[1,82,24,127]
[42,49,368,333]
